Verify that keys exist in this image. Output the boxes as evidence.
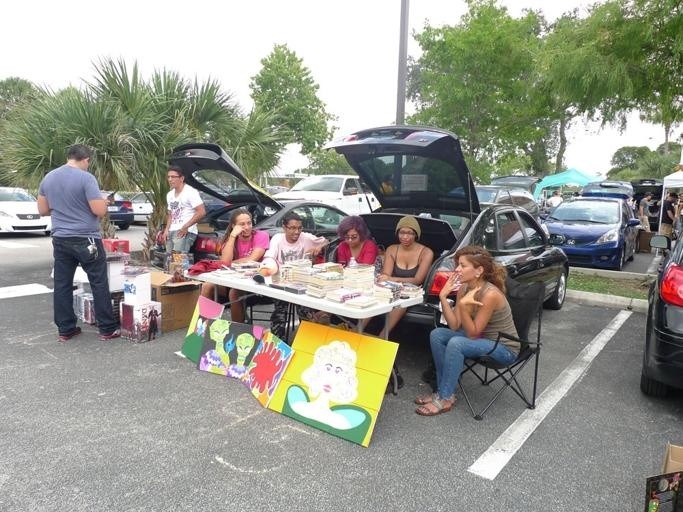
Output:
[90,244,99,261]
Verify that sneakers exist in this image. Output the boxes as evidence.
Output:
[98,330,120,341]
[59,327,81,341]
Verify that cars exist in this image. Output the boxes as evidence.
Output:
[0,187,51,237]
[639,206,681,403]
[128,192,155,225]
[320,126,568,329]
[538,180,640,271]
[471,176,542,220]
[98,191,133,230]
[264,175,380,215]
[631,179,664,232]
[149,143,349,274]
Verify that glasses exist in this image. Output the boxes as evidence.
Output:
[345,236,357,241]
[286,226,304,230]
[167,175,181,179]
[399,231,414,235]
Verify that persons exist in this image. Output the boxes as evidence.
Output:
[352,216,434,341]
[200,210,268,322]
[36,144,120,341]
[330,216,381,335]
[265,213,330,342]
[413,245,524,416]
[638,191,656,232]
[158,167,206,271]
[146,307,158,340]
[542,189,565,209]
[378,174,399,194]
[657,191,682,242]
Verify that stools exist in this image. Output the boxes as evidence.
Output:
[248,295,295,346]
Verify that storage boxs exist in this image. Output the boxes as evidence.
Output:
[145,269,203,339]
[121,265,151,302]
[660,441,683,475]
[118,300,165,344]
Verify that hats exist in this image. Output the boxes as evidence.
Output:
[395,216,421,241]
[645,192,654,195]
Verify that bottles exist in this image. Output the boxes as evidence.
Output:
[347,256,358,267]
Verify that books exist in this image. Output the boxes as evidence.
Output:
[221,257,424,310]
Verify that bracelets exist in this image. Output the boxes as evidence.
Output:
[230,233,236,238]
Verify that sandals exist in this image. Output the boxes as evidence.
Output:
[416,393,456,404]
[416,399,451,415]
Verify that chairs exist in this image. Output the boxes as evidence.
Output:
[425,278,547,420]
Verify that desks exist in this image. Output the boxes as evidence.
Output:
[186,260,423,396]
